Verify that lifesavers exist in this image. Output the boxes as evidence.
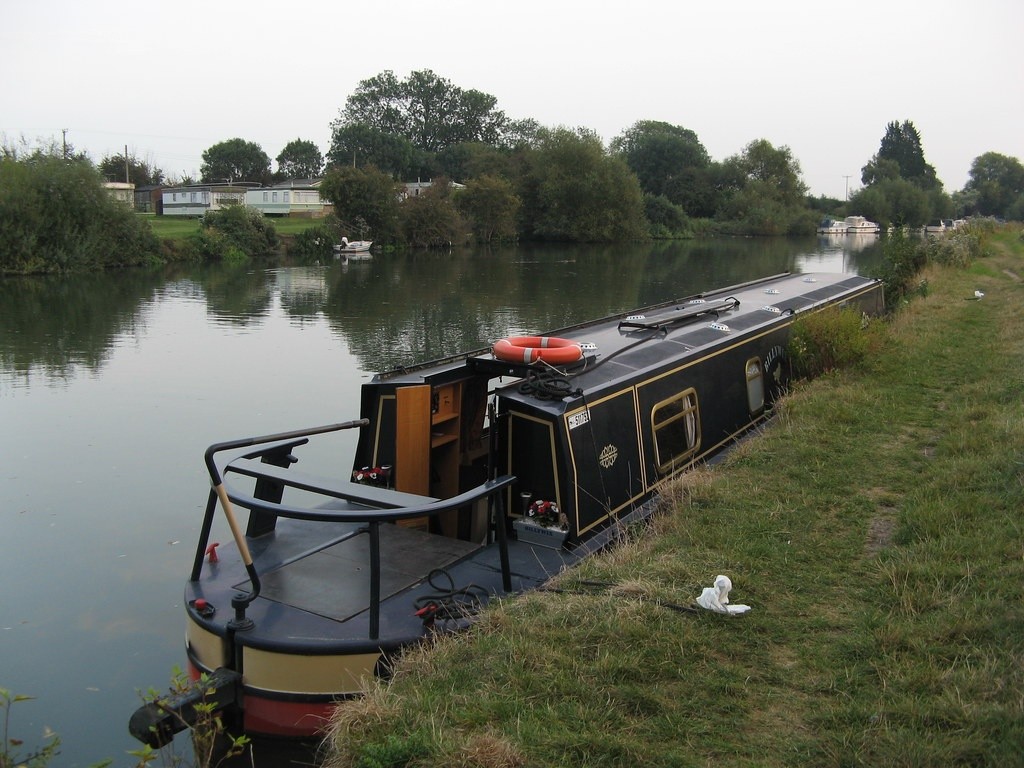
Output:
[492,335,586,365]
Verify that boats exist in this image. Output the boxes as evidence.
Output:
[817,220,848,233]
[846,217,876,233]
[127,270,885,768]
[334,237,374,252]
[927,219,966,232]
